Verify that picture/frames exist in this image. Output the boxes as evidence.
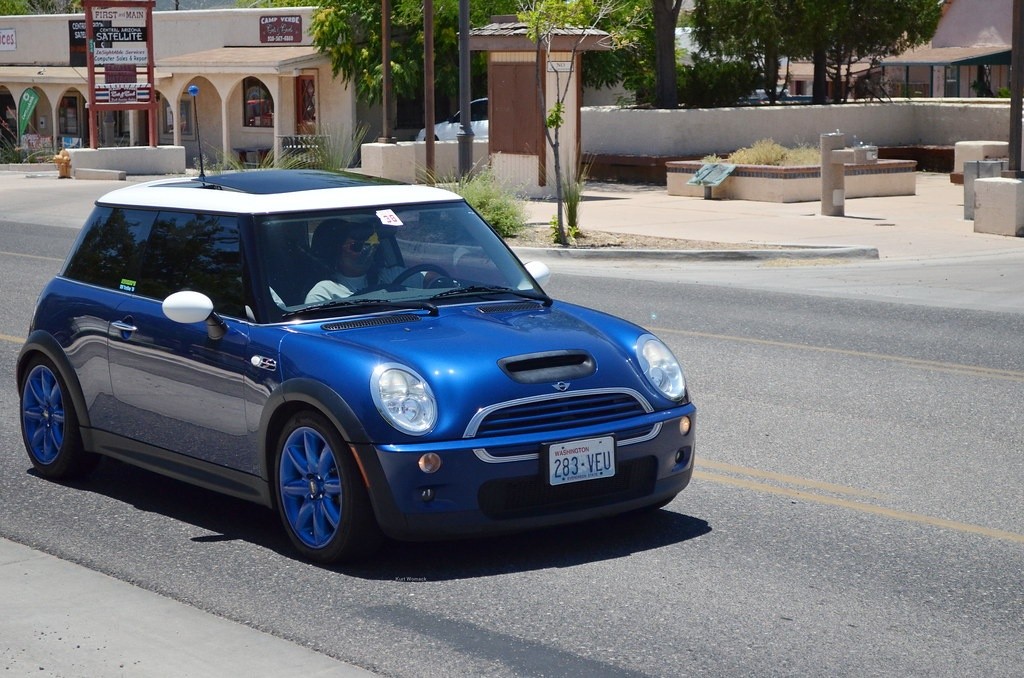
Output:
[158,92,196,141]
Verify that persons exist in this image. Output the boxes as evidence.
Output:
[303,218,460,305]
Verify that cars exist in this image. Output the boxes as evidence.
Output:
[416,97,489,143]
[14,168,697,566]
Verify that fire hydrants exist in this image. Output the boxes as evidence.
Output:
[53,146,73,179]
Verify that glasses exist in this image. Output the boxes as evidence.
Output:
[341,242,377,252]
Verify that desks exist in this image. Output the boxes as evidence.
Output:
[233,146,272,168]
